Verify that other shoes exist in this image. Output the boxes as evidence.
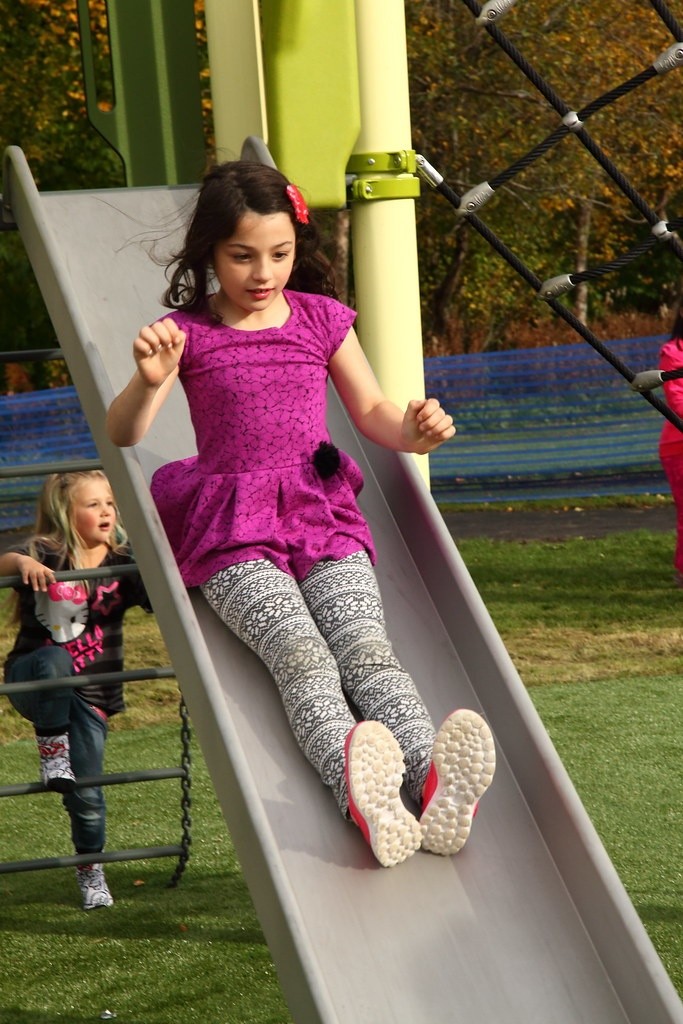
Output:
[418,708,497,856]
[344,721,424,868]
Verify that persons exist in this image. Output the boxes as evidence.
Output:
[658,303,683,584]
[104,160,498,871]
[0,469,157,910]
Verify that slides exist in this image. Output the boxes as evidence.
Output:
[6,135,683,1024]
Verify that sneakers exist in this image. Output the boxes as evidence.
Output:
[32,722,77,791]
[75,853,115,911]
